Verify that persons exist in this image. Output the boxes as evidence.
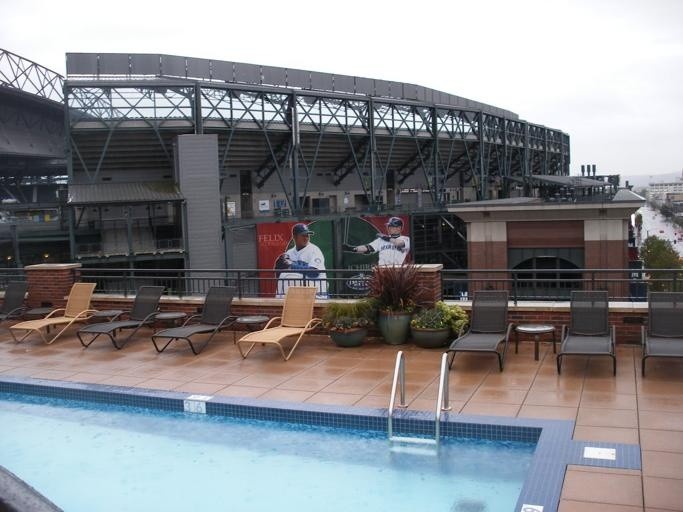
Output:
[274,223,328,298]
[349,216,410,268]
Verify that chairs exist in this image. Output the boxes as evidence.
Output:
[638,290,682,380]
[236,286,321,367]
[441,289,522,376]
[148,288,239,357]
[76,284,170,354]
[7,278,96,346]
[554,288,620,380]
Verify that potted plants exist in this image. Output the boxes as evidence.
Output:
[322,298,378,351]
[368,258,431,347]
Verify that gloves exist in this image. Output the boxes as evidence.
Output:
[342,242,357,253]
[377,232,391,243]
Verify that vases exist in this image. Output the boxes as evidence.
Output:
[409,322,450,352]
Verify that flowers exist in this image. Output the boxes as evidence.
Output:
[410,296,468,339]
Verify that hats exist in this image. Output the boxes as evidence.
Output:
[292,223,314,237]
[385,217,404,229]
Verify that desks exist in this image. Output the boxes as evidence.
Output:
[511,322,556,363]
[235,316,269,334]
[153,309,188,330]
[85,309,122,339]
[27,305,61,336]
